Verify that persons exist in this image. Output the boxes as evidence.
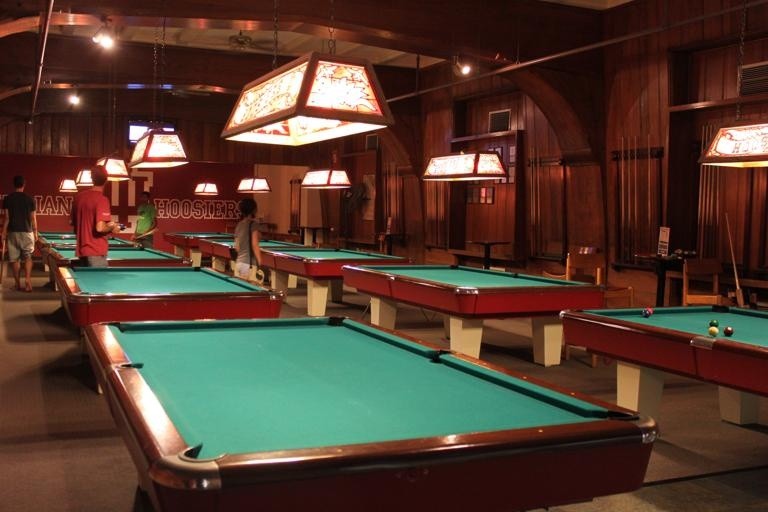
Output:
[131,191,159,249]
[234,198,265,285]
[2,175,38,293]
[68,165,121,267]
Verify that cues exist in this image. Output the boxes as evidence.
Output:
[724,211,745,309]
[696,124,720,260]
[620,134,652,265]
[529,145,545,258]
[136,226,160,239]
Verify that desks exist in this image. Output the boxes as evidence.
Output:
[34,231,768,510]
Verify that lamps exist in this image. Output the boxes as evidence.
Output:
[58,179,78,192]
[76,169,94,186]
[421,152,508,181]
[301,169,352,189]
[92,15,115,48]
[194,183,218,196]
[700,117,768,170]
[238,178,271,193]
[96,51,130,181]
[128,29,190,169]
[68,90,79,105]
[221,5,395,147]
[452,57,473,77]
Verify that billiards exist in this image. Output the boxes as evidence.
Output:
[708,318,734,335]
[51,241,55,245]
[61,235,65,240]
[642,307,654,316]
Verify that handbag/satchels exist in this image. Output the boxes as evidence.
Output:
[248,266,265,285]
